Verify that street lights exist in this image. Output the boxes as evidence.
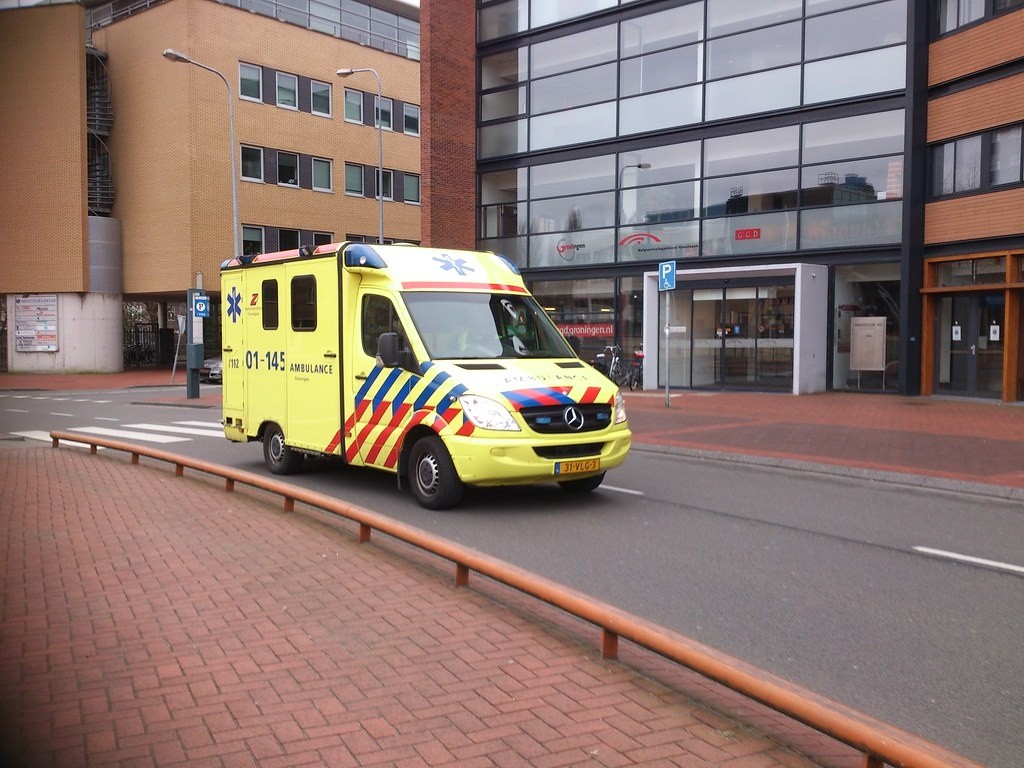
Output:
[609,163,652,384]
[335,68,384,243]
[162,47,239,258]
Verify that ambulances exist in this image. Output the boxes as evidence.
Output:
[217,240,637,511]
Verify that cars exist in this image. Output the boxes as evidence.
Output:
[199,354,222,385]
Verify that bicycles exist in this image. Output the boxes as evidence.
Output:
[588,343,645,392]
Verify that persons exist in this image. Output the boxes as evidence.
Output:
[394,316,409,352]
